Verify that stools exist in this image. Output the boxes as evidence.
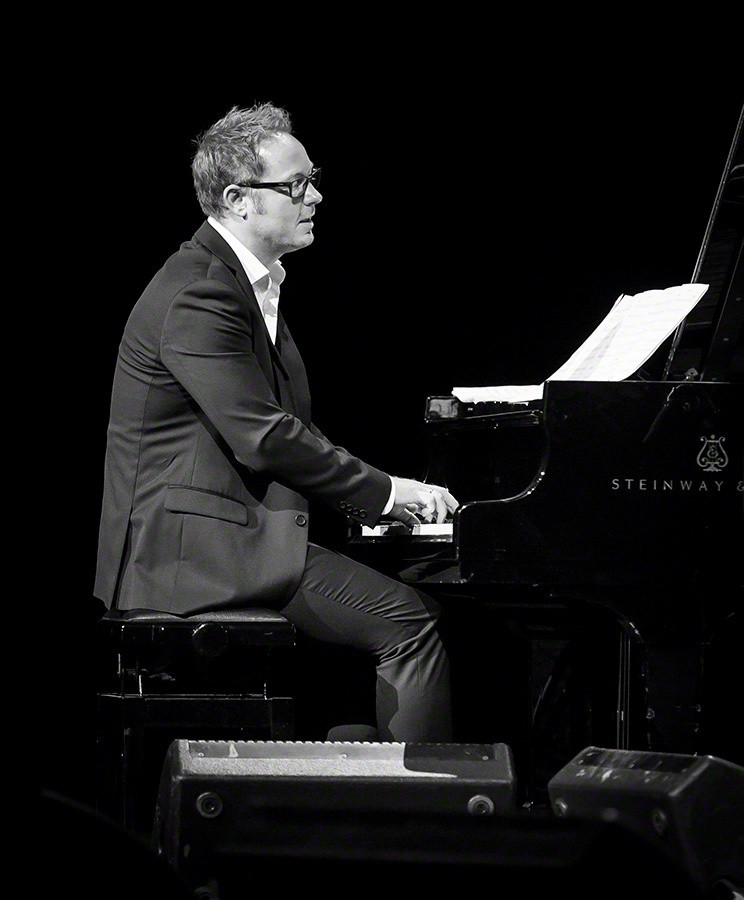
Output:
[102,607,299,836]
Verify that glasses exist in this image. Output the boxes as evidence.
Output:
[237,168,322,198]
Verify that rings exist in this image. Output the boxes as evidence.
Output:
[429,488,433,493]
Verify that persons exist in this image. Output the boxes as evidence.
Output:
[92,102,460,743]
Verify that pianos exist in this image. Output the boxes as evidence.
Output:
[340,368,744,759]
